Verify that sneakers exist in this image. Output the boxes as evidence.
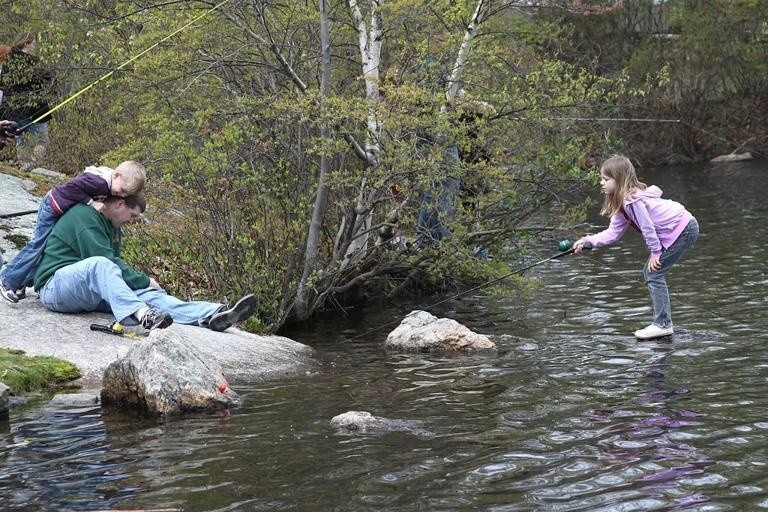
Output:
[0,279,28,303]
[208,293,257,332]
[634,324,674,339]
[139,307,174,338]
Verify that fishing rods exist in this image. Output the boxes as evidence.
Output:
[90,322,142,342]
[329,238,592,351]
[462,116,682,123]
[0,0,229,148]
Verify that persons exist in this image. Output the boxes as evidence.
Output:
[36,404,182,509]
[0,160,149,304]
[1,29,60,174]
[566,152,699,341]
[411,87,496,250]
[583,344,716,512]
[31,188,258,333]
[0,118,26,145]
